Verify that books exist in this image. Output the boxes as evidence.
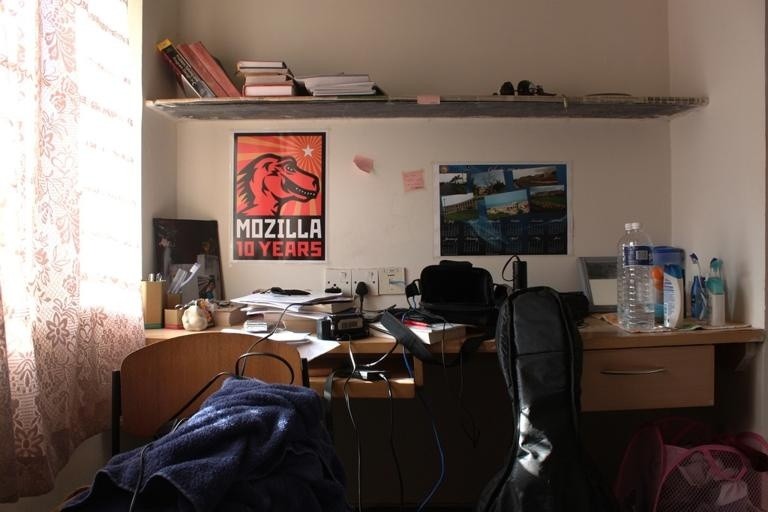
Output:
[155,38,377,100]
[309,362,338,378]
[365,318,467,346]
[210,294,355,328]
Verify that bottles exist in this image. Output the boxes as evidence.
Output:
[616,221,656,333]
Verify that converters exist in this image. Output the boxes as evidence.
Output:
[343,367,392,381]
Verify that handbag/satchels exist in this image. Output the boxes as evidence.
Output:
[417,259,496,325]
[614,414,768,512]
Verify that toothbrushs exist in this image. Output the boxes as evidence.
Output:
[175,262,202,294]
[689,252,707,306]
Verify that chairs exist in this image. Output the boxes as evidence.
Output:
[110,332,304,457]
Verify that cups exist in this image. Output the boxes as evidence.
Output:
[695,285,711,325]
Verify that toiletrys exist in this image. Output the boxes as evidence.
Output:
[663,264,684,328]
[706,278,725,326]
[690,275,700,318]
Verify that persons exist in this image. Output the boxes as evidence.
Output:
[199,279,217,298]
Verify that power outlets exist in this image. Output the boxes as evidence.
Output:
[324,267,379,296]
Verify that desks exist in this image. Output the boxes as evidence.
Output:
[145,317,765,398]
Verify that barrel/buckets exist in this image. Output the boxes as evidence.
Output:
[617,223,654,332]
